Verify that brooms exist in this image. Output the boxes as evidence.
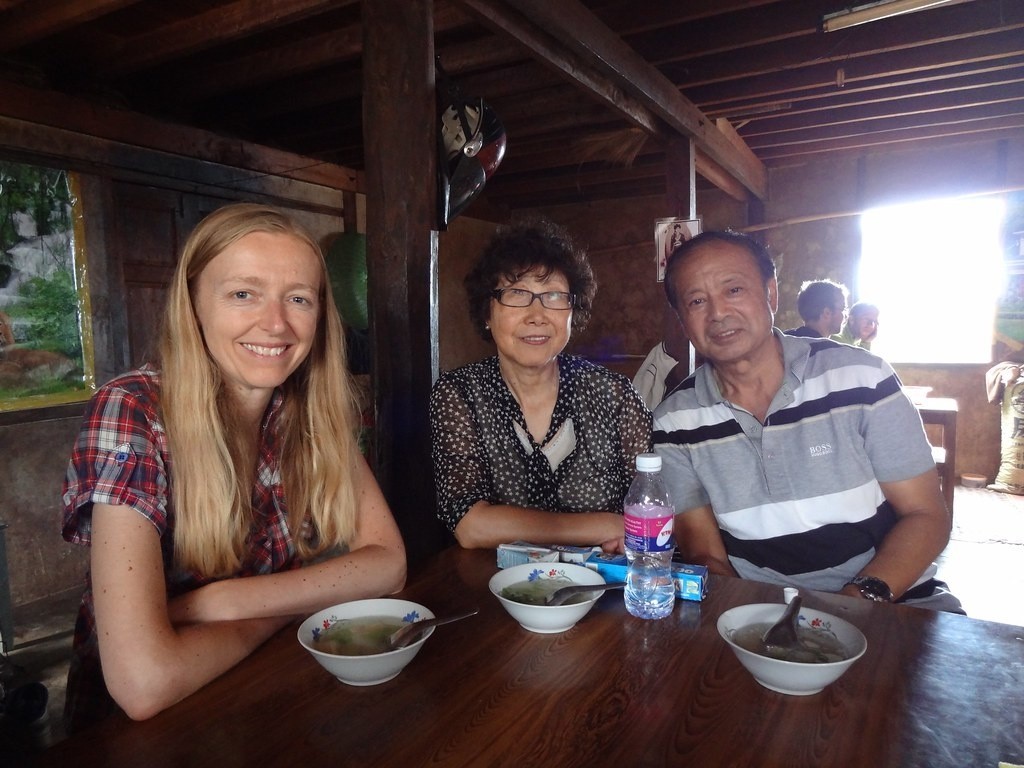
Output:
[574,101,792,170]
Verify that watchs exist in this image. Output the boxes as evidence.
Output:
[843,576,893,605]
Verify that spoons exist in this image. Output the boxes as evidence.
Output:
[546,582,627,606]
[384,607,480,651]
[761,596,803,648]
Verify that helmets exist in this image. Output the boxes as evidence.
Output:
[439,95,507,232]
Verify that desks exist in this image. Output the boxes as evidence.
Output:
[912,398,958,530]
[3,543,1024,768]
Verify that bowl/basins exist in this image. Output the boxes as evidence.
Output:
[898,387,932,404]
[489,563,606,634]
[296,598,438,687]
[716,603,868,696]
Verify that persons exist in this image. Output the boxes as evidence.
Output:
[784,279,850,339]
[428,220,651,556]
[652,230,971,614]
[61,205,409,731]
[830,301,886,359]
[671,224,686,252]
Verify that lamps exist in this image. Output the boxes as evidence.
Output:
[816,0,977,36]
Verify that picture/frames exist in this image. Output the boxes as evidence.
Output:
[655,218,701,283]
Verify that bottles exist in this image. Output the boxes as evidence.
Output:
[623,453,676,620]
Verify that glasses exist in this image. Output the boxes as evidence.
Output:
[835,304,850,315]
[489,288,576,311]
